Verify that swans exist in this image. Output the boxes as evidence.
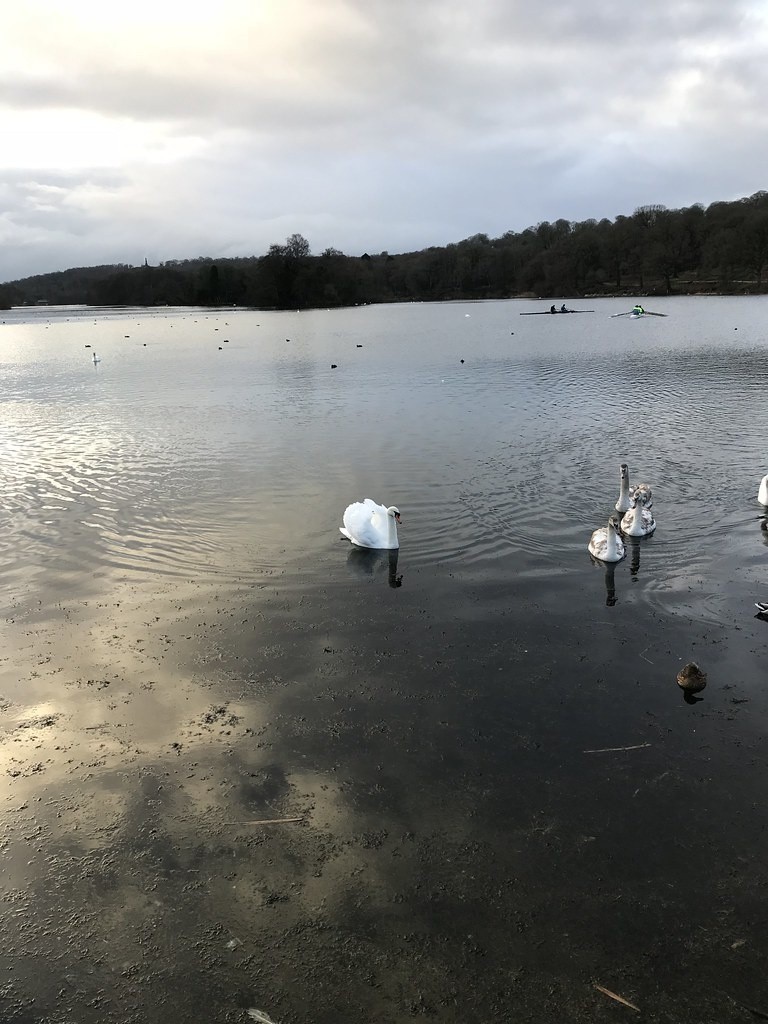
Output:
[339,498,402,550]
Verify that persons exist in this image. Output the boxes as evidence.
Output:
[551,305,557,313]
[633,304,645,315]
[560,304,567,311]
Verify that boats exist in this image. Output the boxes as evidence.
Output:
[630,306,645,318]
[519,310,596,315]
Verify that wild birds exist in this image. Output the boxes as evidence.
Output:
[676,662,707,694]
[587,464,657,562]
[757,474,768,532]
[92,352,101,362]
[754,601,768,616]
